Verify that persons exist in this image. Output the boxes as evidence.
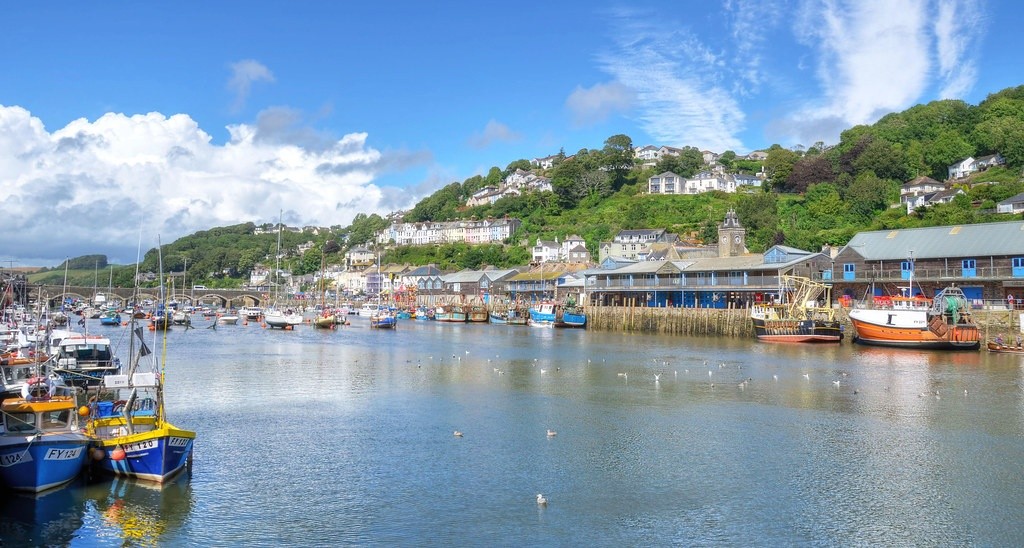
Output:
[998,334,1003,344]
[958,312,971,324]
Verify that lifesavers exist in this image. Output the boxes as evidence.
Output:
[20,376,57,403]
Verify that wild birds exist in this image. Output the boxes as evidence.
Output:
[587,358,592,362]
[854,389,860,394]
[675,369,691,375]
[772,374,778,382]
[711,383,715,387]
[843,372,850,376]
[936,391,941,394]
[919,393,927,397]
[885,387,892,392]
[738,377,752,386]
[964,390,969,394]
[453,431,462,436]
[546,429,557,436]
[603,359,607,362]
[803,374,810,378]
[704,360,709,365]
[536,494,548,504]
[618,373,629,378]
[654,373,661,381]
[719,361,727,367]
[353,351,560,376]
[832,381,840,386]
[708,371,713,377]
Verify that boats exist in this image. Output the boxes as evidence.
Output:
[0,474,102,546]
[848,271,982,350]
[104,467,197,512]
[987,341,1023,352]
[527,307,586,329]
[750,275,845,343]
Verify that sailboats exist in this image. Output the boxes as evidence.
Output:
[0,207,529,496]
